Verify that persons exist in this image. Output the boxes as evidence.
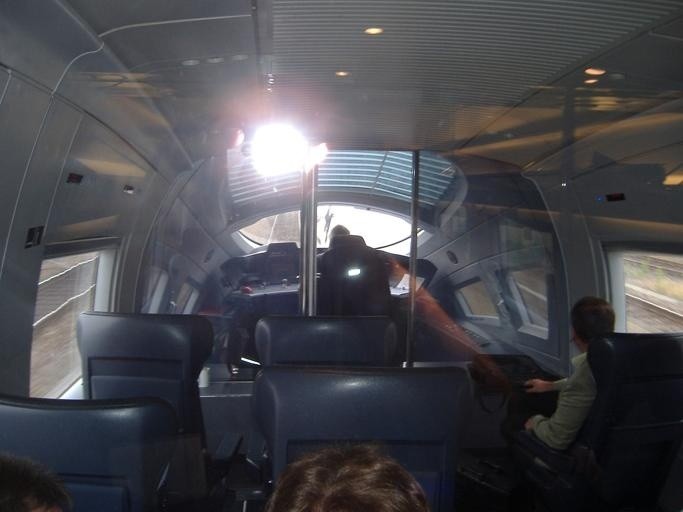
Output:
[0,453,73,512]
[524,295,617,451]
[317,224,350,273]
[264,442,429,512]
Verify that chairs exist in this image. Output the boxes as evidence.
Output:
[502,332,683,511]
[75,310,213,460]
[255,315,400,369]
[0,392,183,512]
[318,234,388,319]
[252,365,474,512]
